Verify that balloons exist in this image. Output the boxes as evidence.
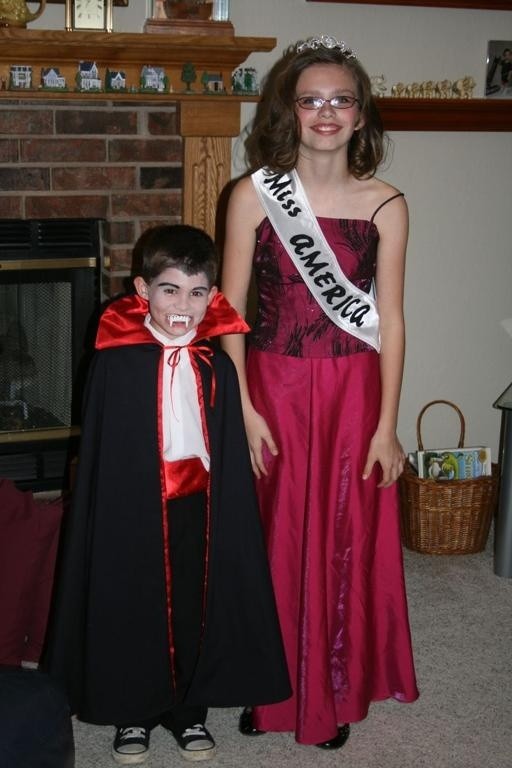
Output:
[65,0,113,33]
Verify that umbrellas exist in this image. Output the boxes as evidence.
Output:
[314,719,351,750]
[238,705,267,737]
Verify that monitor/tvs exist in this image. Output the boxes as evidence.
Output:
[418,447,491,480]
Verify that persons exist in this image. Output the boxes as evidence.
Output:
[39,226,292,765]
[214,37,419,749]
[497,49,512,84]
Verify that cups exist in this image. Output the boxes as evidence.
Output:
[293,96,362,111]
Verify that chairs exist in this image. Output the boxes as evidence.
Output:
[167,724,217,760]
[111,721,152,764]
